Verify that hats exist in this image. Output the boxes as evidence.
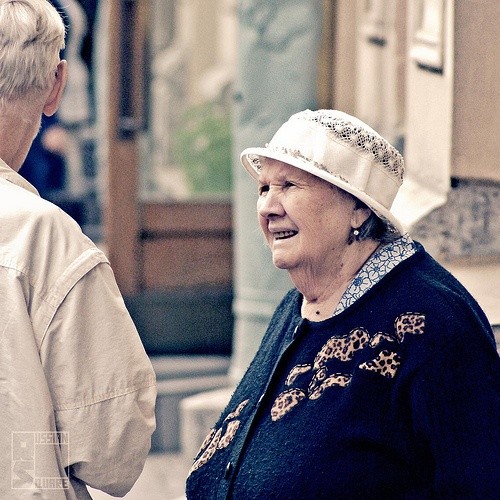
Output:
[240,109,406,237]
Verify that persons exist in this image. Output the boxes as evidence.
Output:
[0,0,159,500]
[184,110,500,500]
